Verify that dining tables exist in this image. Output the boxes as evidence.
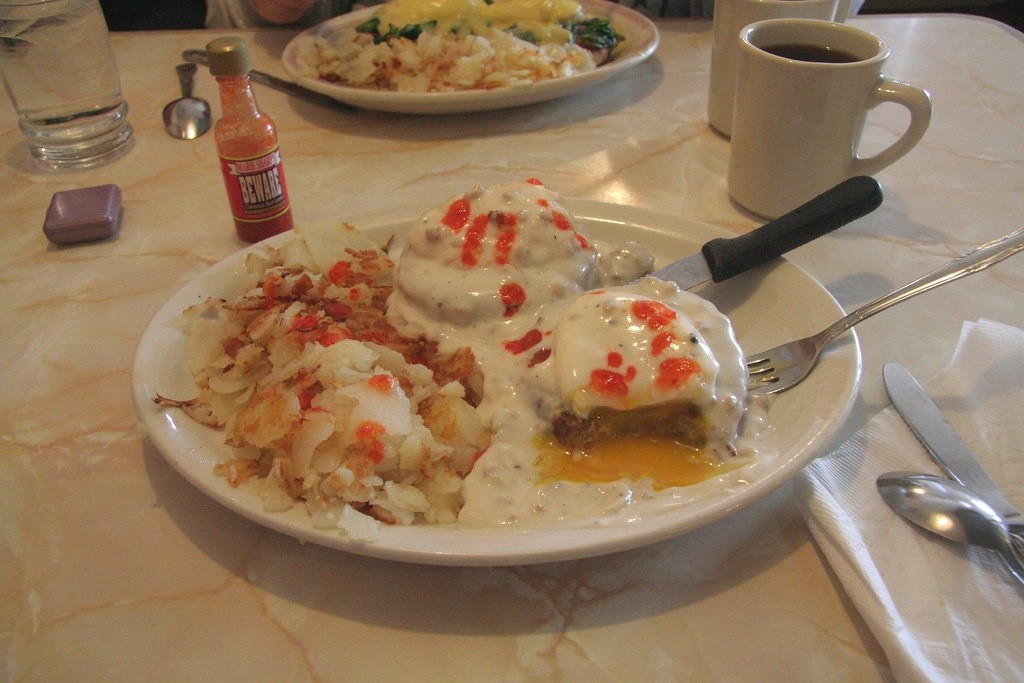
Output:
[0,11,1024,683]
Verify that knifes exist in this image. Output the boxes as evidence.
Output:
[623,175,884,291]
[182,48,362,115]
[882,362,1024,559]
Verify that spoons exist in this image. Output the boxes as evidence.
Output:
[162,63,212,140]
[876,470,1024,589]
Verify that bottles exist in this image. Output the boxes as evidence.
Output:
[206,36,295,242]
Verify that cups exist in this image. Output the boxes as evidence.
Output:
[707,0,867,136]
[0,0,136,173]
[728,17,933,219]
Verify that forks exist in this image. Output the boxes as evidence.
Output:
[746,227,1024,395]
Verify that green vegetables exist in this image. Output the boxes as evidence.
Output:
[354,12,620,51]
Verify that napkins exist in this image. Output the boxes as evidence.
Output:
[794,317,1024,683]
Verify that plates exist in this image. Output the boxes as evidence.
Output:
[281,0,661,115]
[132,194,862,565]
